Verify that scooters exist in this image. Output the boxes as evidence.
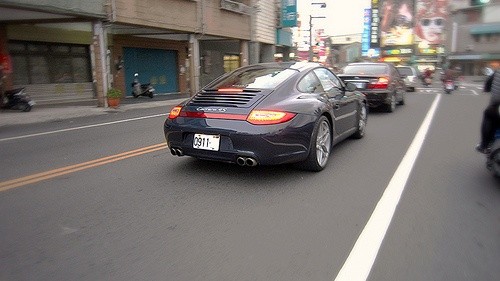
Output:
[130,72,155,98]
[481,66,500,177]
[443,80,455,94]
[0,76,35,112]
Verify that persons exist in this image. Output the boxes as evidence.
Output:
[476,66,500,153]
[416,0,449,46]
[421,65,458,88]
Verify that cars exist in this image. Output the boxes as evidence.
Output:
[394,64,418,92]
[335,62,406,112]
[163,61,368,171]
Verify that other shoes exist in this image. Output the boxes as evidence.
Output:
[476,144,490,154]
